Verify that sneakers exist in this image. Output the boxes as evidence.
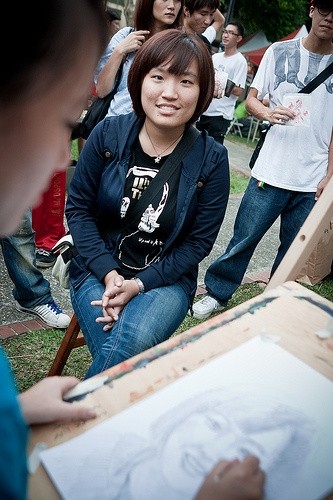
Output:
[36,247,55,267]
[14,298,72,328]
[188,296,223,319]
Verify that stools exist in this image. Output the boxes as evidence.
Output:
[47,313,86,377]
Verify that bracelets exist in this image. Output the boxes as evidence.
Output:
[132,277,144,295]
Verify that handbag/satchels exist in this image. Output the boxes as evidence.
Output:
[51,232,80,290]
[249,124,273,169]
[79,91,115,140]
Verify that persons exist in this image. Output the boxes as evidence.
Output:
[66,29,230,382]
[183,0,220,35]
[105,11,121,34]
[196,22,248,144]
[198,8,226,54]
[0,0,267,500]
[32,170,66,266]
[188,0,333,319]
[0,204,71,328]
[95,0,184,116]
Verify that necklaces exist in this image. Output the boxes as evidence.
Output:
[144,123,184,164]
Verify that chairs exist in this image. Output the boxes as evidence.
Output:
[226,117,243,138]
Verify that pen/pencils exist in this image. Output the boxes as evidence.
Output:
[215,459,238,480]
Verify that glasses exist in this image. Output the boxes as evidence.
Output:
[222,30,240,36]
[313,4,333,18]
